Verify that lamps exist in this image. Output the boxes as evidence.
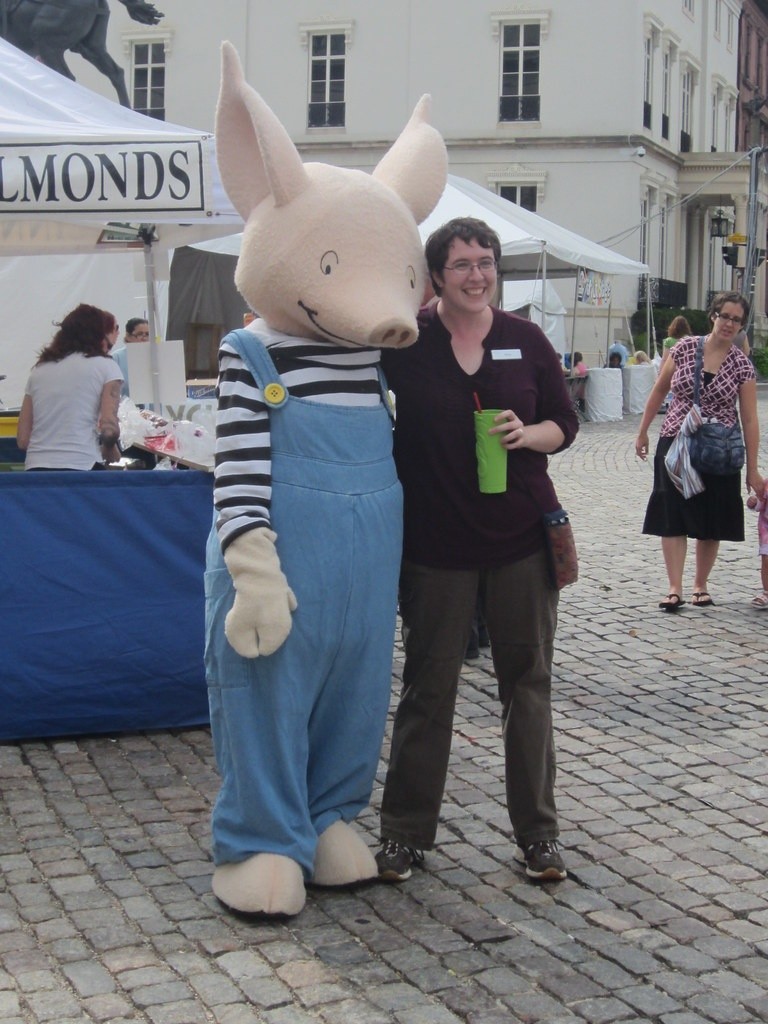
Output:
[710,193,729,237]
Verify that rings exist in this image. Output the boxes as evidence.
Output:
[519,428,523,436]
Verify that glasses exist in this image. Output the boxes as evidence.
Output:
[715,312,743,325]
[129,332,149,339]
[104,335,112,350]
[443,259,498,276]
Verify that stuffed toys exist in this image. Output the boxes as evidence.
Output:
[204,40,448,915]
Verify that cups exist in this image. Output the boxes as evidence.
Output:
[474,410,508,493]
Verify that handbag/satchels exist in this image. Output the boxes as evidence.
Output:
[542,509,578,589]
[690,420,745,475]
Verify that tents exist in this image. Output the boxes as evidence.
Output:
[0,36,246,418]
[170,175,657,377]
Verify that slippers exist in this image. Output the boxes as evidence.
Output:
[658,594,686,607]
[692,592,712,605]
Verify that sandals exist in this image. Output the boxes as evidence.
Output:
[750,591,768,609]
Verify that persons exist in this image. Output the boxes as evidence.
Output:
[604,352,622,368]
[16,305,124,471]
[733,330,750,356]
[747,479,768,608]
[376,217,580,879]
[635,290,765,608]
[106,311,149,397]
[635,351,649,365]
[557,352,586,411]
[660,316,692,373]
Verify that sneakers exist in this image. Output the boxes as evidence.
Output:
[513,839,567,879]
[374,836,425,879]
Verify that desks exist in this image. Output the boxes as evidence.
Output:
[116,436,217,472]
